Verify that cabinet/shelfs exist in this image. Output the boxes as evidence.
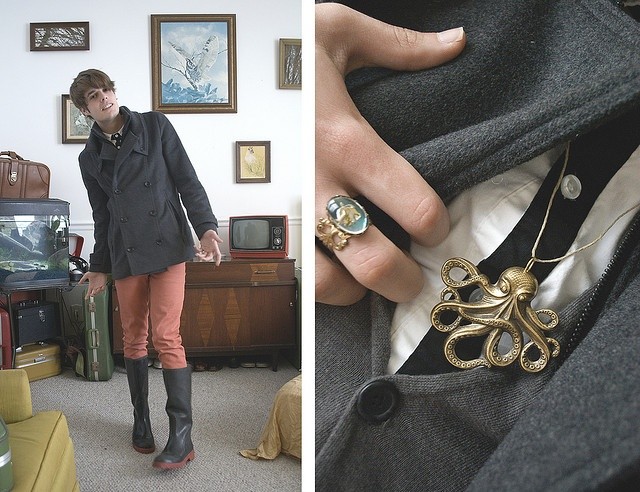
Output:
[113,254,296,371]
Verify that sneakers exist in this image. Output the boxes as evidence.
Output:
[195,356,208,372]
[154,356,162,369]
[256,356,271,368]
[206,357,224,371]
[241,357,255,368]
[147,358,154,367]
[186,357,195,371]
[226,356,240,368]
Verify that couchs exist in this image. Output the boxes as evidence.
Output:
[1,369,80,491]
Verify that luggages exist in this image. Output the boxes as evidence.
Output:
[0,151,51,199]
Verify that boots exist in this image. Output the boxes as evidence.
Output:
[152,363,194,469]
[123,352,157,454]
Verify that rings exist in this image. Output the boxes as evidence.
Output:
[317,194,372,250]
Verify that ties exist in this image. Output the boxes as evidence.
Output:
[111,133,122,150]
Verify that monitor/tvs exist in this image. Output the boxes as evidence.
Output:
[228,215,289,258]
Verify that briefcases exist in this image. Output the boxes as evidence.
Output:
[83,285,115,382]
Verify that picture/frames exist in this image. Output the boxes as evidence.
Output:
[150,14,238,114]
[235,141,272,184]
[278,38,302,91]
[29,21,90,51]
[61,93,95,144]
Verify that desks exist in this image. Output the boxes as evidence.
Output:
[0,281,87,369]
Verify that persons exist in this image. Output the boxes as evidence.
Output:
[315,0,640,492]
[70,70,223,469]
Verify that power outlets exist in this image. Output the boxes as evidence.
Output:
[70,304,84,322]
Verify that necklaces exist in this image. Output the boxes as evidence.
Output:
[430,144,639,375]
